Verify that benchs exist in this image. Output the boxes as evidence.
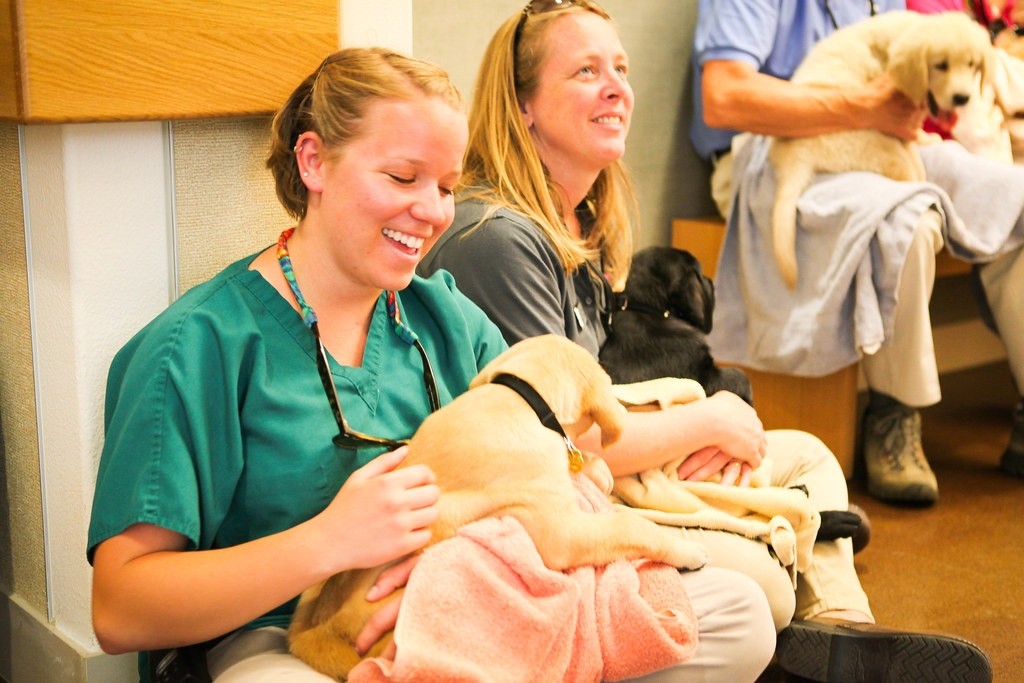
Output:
[672,213,1009,483]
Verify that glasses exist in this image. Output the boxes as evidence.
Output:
[310,321,440,452]
[514,0,574,47]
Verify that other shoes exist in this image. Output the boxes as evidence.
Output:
[776,619,993,683]
[848,504,870,554]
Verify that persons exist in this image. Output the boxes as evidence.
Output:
[696,0,1024,507]
[89,0,1002,683]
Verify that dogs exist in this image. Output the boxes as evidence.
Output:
[596,245,860,543]
[287,333,708,683]
[767,10,995,292]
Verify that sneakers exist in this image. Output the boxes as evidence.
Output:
[999,397,1024,477]
[860,391,938,506]
